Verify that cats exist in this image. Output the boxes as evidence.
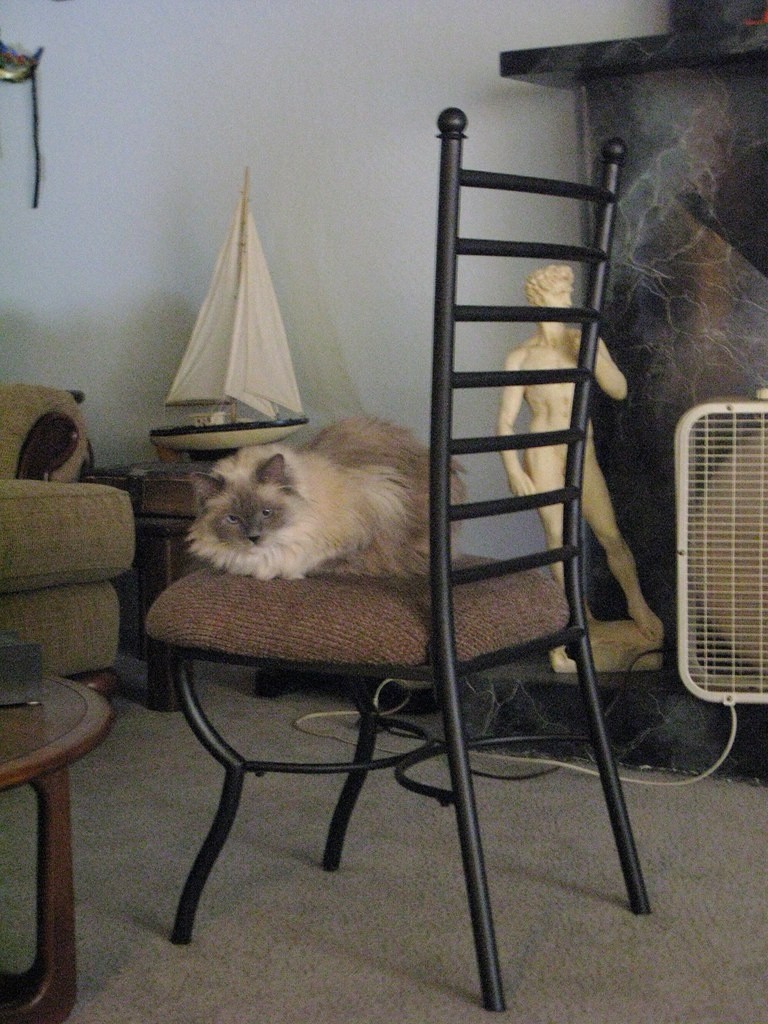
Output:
[180,411,471,584]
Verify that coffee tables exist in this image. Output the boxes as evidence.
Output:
[0,676,116,1024]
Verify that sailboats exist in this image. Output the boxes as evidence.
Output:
[149,164,310,449]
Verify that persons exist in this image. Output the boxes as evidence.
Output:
[500,267,664,646]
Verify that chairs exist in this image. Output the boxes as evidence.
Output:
[144,105,653,1013]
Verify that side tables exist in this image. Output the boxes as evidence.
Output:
[85,473,285,712]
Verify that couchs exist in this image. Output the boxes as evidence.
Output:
[0,382,135,703]
